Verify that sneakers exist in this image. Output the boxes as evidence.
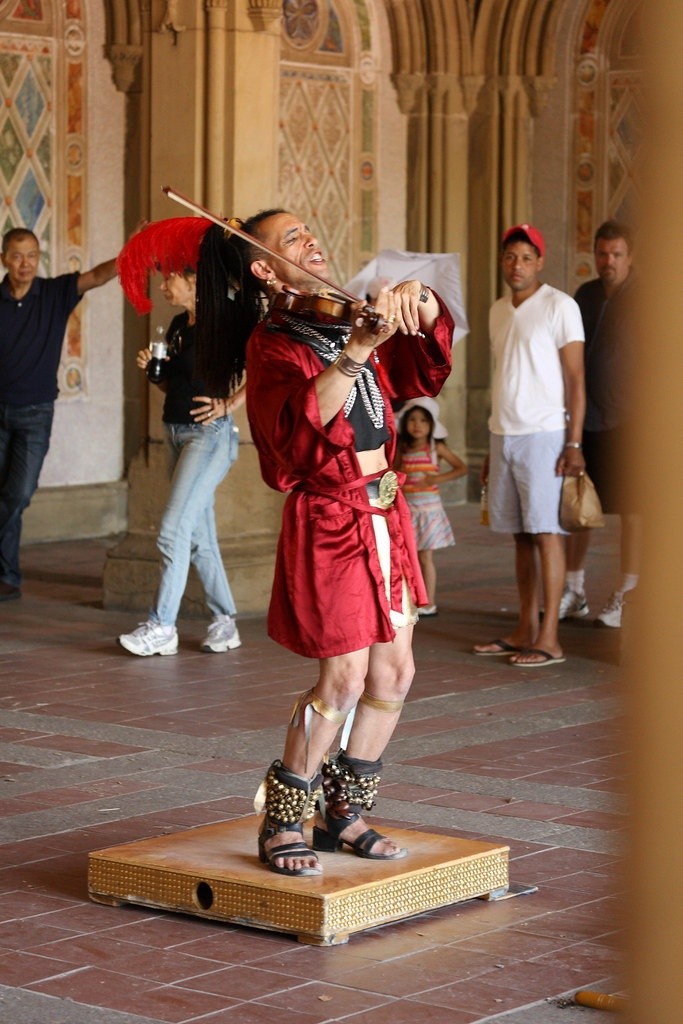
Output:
[200,614,241,653]
[539,584,589,623]
[593,597,621,628]
[115,619,179,656]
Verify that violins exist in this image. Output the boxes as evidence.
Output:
[268,292,392,337]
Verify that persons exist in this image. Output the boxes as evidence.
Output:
[538,220,639,629]
[391,397,470,615]
[0,228,117,601]
[118,217,246,657]
[240,207,454,877]
[470,223,586,669]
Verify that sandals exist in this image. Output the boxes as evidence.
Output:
[313,798,408,860]
[259,815,322,876]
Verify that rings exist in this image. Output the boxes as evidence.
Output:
[208,412,211,418]
[579,472,584,476]
[387,315,395,324]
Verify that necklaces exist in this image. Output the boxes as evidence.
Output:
[8,280,26,307]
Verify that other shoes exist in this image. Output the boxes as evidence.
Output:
[417,605,438,616]
[0,582,22,603]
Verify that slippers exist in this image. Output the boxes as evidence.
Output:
[473,638,522,656]
[510,647,566,667]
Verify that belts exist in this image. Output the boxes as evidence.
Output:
[365,471,399,506]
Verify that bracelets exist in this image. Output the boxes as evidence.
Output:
[563,441,583,451]
[419,281,430,304]
[334,351,364,378]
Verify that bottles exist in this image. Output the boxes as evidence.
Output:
[479,479,489,525]
[145,325,167,383]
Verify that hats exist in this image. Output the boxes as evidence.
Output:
[504,223,545,258]
[393,396,450,439]
